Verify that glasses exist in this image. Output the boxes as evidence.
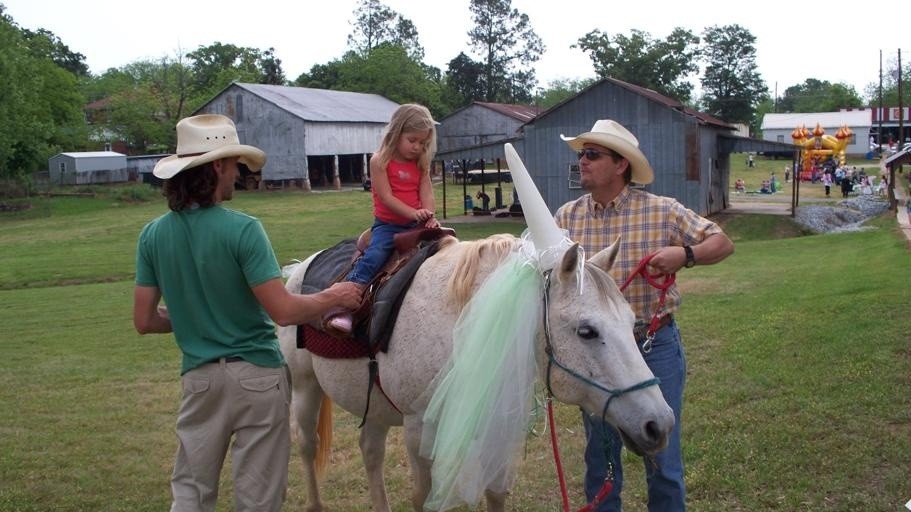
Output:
[578,148,613,161]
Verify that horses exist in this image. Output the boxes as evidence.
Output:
[269,232,679,512]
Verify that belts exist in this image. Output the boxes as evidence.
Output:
[636,314,670,339]
[209,356,242,364]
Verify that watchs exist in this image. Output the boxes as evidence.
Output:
[684,246,695,268]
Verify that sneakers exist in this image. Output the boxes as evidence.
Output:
[326,310,354,333]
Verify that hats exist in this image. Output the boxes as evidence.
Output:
[153,113,266,179]
[560,119,654,184]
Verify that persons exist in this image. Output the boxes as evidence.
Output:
[733,133,900,199]
[475,191,490,211]
[552,119,735,512]
[330,103,442,334]
[132,114,363,512]
